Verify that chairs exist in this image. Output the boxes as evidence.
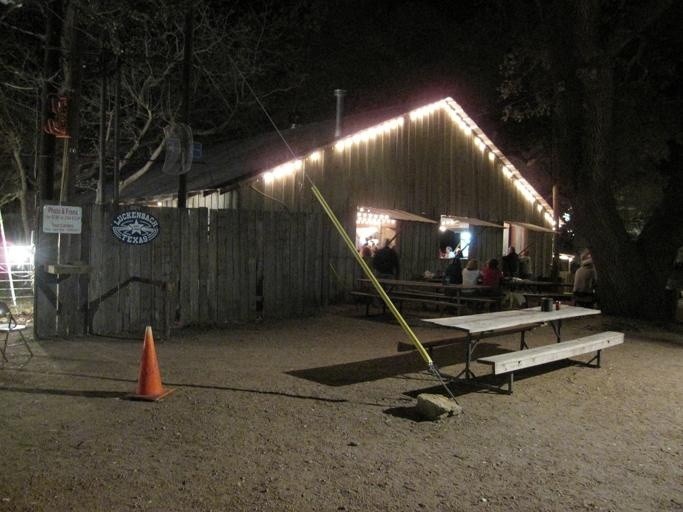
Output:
[0,302,33,362]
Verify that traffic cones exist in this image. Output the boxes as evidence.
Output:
[125,326,176,404]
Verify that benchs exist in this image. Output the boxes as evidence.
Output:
[348,278,598,317]
[477,331,624,393]
[398,323,548,356]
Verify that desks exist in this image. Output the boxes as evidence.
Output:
[419,303,601,391]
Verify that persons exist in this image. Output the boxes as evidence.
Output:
[438,246,532,313]
[372,239,400,304]
[573,248,597,308]
